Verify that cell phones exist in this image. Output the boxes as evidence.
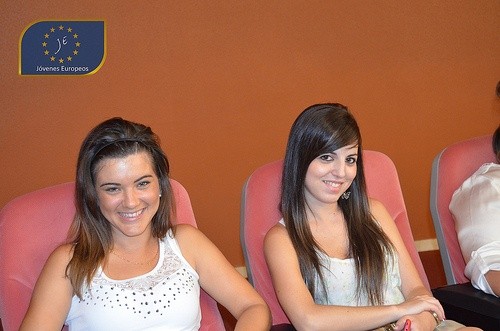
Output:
[403,319,411,331]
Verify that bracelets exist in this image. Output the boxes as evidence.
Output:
[431,311,441,328]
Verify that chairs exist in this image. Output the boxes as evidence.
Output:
[429,133,500,331]
[0,177,226,331]
[240,150,434,331]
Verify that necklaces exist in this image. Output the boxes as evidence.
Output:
[110,249,158,267]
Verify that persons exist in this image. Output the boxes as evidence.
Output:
[449,128,500,299]
[263,103,483,331]
[17,117,271,331]
